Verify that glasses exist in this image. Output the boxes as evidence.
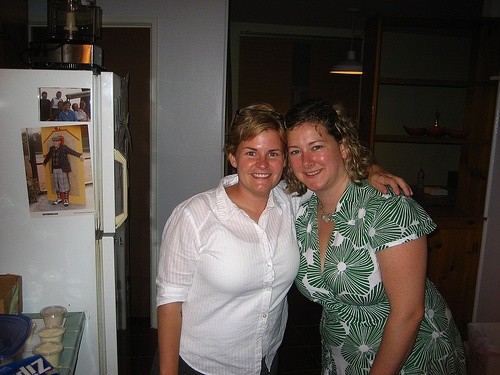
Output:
[231,108,284,131]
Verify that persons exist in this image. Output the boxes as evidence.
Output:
[284,98,465,375]
[41,91,90,121]
[155,104,413,375]
[42,136,84,207]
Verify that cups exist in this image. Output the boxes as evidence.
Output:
[32,305,67,366]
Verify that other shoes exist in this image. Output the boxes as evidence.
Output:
[63,198,69,206]
[53,198,62,205]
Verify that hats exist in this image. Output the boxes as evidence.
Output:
[52,137,60,141]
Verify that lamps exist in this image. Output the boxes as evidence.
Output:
[330,8,363,75]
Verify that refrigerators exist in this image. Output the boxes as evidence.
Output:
[0,69,131,375]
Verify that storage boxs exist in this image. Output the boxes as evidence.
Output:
[0,275,23,316]
[0,314,34,366]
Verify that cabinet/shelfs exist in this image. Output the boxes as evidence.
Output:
[359,12,500,341]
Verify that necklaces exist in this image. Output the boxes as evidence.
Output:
[318,199,333,224]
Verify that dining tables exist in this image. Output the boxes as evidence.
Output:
[21,312,86,375]
